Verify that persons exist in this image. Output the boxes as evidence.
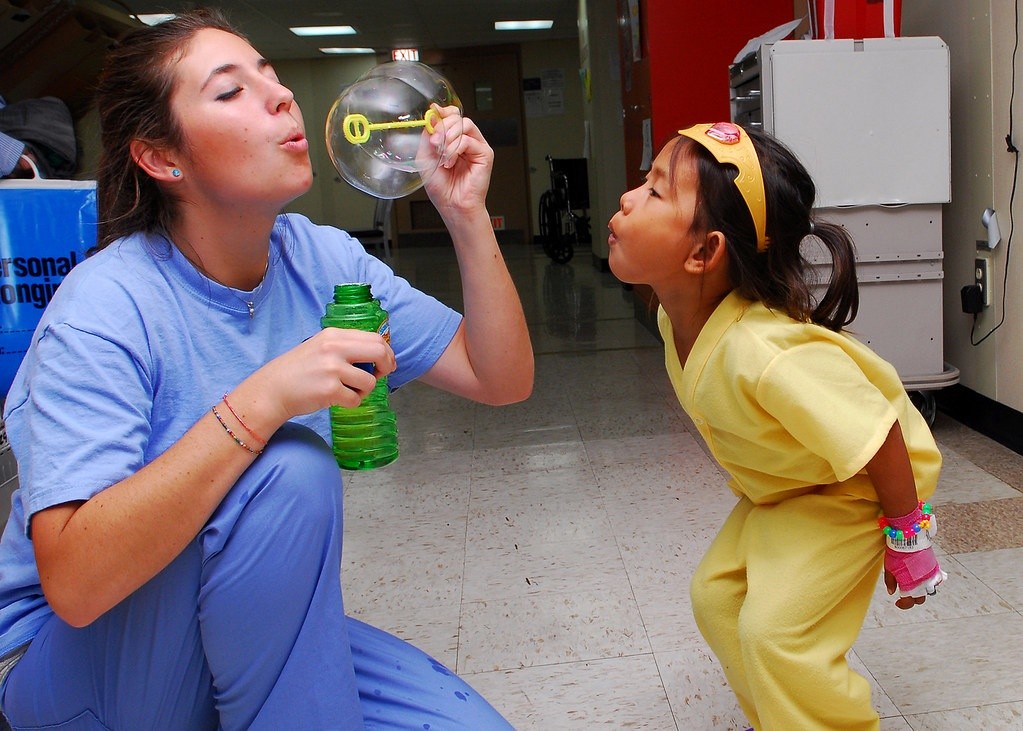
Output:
[608,122,947,731]
[0,18,534,731]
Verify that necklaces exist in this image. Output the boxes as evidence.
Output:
[182,248,270,319]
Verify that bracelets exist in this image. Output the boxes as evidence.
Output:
[212,394,267,454]
[878,497,932,540]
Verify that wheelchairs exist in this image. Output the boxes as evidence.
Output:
[537,154,589,264]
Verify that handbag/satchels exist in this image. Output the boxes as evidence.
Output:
[0,154,100,399]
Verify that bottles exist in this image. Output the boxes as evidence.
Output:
[320,282,401,471]
